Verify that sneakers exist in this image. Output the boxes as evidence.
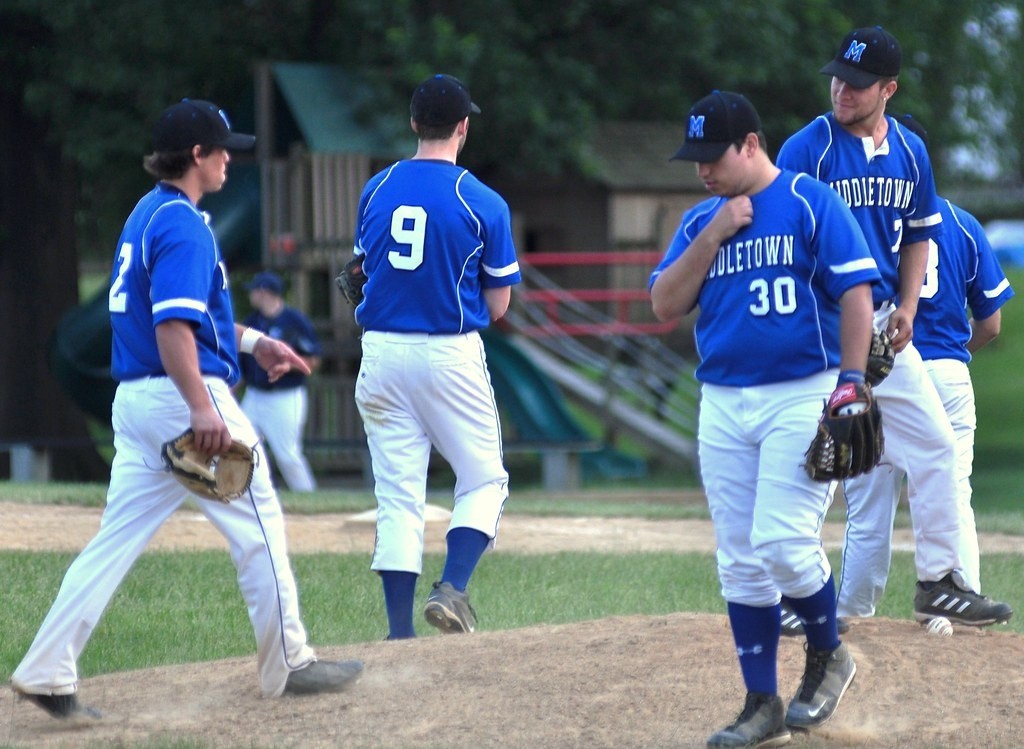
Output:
[424,579,479,635]
[786,640,856,728]
[914,579,1013,627]
[778,608,850,636]
[707,692,790,749]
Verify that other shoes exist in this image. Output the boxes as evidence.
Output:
[11,678,103,724]
[284,660,365,693]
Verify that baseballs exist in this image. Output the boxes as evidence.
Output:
[926,616,954,636]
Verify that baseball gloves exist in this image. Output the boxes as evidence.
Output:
[865,330,896,388]
[162,427,256,503]
[803,382,886,483]
[334,252,367,307]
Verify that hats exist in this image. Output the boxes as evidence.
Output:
[669,91,761,162]
[820,26,900,89]
[241,270,284,292]
[889,113,928,145]
[158,99,256,152]
[410,75,483,125]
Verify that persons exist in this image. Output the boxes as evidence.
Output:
[352,71,518,640]
[907,193,1018,594]
[234,269,326,488]
[647,87,879,749]
[5,97,362,719]
[774,24,1016,632]
